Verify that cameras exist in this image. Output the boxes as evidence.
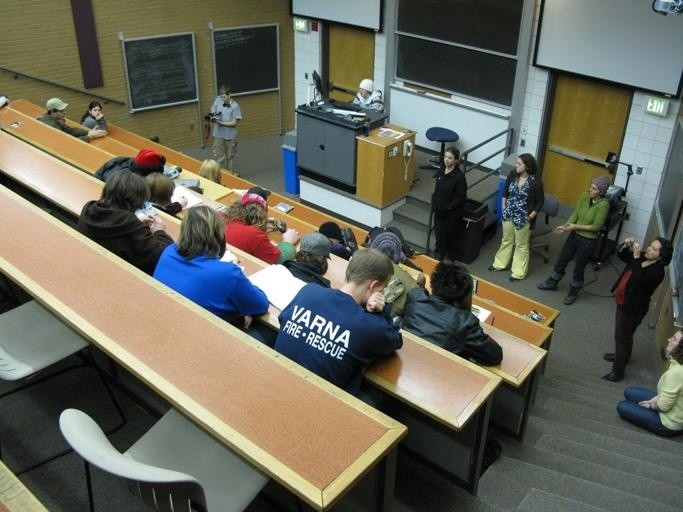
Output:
[205,112,222,122]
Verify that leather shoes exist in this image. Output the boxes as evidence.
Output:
[603,353,630,361]
[601,367,624,382]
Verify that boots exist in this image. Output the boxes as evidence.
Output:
[563,287,579,305]
[536,275,558,290]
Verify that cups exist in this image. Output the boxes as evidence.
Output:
[384,118,390,128]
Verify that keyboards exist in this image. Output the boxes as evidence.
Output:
[331,99,363,113]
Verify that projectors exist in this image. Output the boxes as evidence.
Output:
[653,0,683,16]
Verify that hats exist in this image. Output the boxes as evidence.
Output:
[319,222,342,240]
[371,232,401,265]
[359,79,373,92]
[247,187,270,201]
[592,175,610,195]
[46,98,69,111]
[301,233,331,260]
[129,147,166,170]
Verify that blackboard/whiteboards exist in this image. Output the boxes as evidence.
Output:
[398,0,523,55]
[121,32,199,113]
[652,116,682,241]
[211,23,279,97]
[659,215,683,320]
[396,34,518,108]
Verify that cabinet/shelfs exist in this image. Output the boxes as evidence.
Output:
[356,124,417,209]
[572,202,628,265]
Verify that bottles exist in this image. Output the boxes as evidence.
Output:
[364,121,369,137]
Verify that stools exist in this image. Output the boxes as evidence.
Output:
[420,128,459,178]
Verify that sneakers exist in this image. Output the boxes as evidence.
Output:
[510,275,527,281]
[488,264,508,271]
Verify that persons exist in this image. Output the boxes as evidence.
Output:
[222,193,300,264]
[82,100,106,132]
[284,233,331,289]
[37,97,108,144]
[537,175,610,305]
[209,85,242,174]
[368,232,421,326]
[248,187,286,234]
[602,237,674,382]
[153,206,277,349]
[404,259,502,466]
[617,330,683,437]
[489,154,545,282]
[135,172,175,219]
[95,148,187,214]
[353,78,384,115]
[78,169,174,275]
[274,248,404,479]
[431,146,467,260]
[199,158,221,185]
[319,222,352,261]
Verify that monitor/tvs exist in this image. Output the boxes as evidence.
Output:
[311,70,325,97]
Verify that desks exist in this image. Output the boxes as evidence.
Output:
[0,460,50,512]
[0,130,502,498]
[1,106,548,443]
[7,98,554,375]
[93,123,562,326]
[1,186,409,512]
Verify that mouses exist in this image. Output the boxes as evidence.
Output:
[328,97,335,103]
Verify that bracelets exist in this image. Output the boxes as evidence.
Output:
[528,216,533,221]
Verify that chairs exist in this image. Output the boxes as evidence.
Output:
[59,405,269,512]
[1,300,126,475]
[529,195,558,264]
